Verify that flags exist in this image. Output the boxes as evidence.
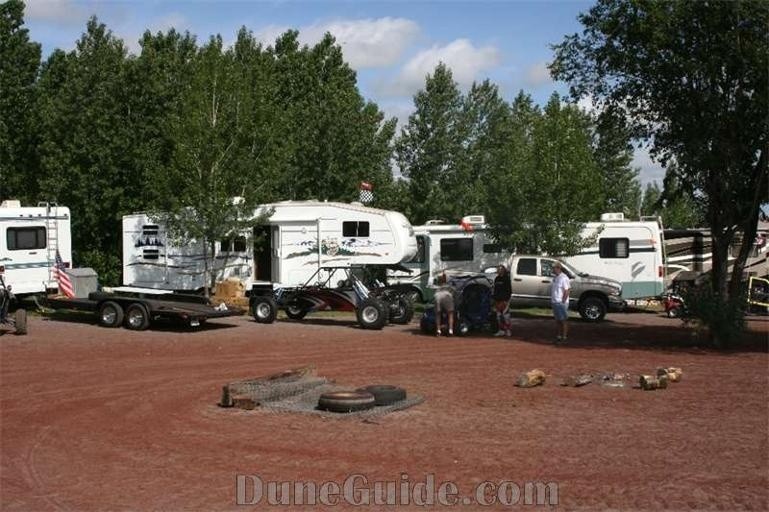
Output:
[359,190,373,202]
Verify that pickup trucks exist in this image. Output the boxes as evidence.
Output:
[445,254,622,321]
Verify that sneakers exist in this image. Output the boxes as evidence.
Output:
[435,332,453,336]
[495,329,511,336]
[554,336,568,344]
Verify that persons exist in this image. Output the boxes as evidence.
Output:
[434,284,457,337]
[550,261,572,343]
[493,264,512,336]
